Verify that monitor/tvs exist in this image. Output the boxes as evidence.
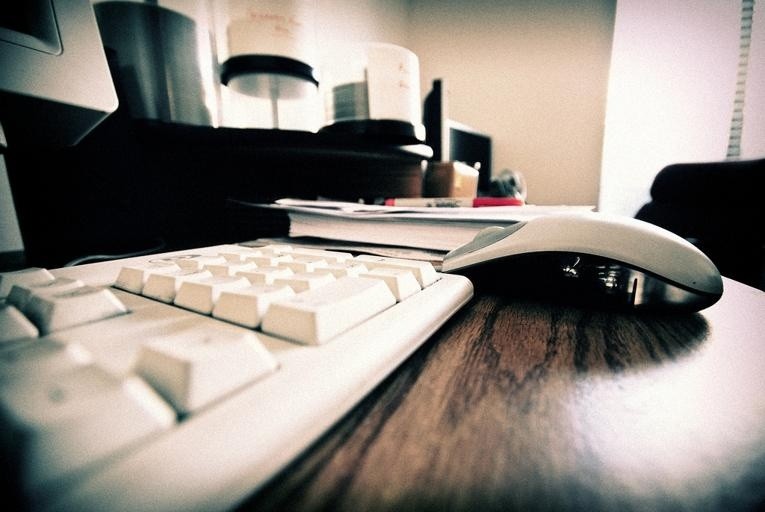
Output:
[0,1,121,264]
[448,119,493,195]
[422,77,450,162]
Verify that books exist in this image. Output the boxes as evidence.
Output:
[276,194,599,273]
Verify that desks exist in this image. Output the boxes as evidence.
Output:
[223,270,765,512]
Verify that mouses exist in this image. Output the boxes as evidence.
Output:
[440,208,722,320]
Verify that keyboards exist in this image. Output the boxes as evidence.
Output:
[0,235,478,512]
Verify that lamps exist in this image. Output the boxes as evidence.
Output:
[221,14,318,132]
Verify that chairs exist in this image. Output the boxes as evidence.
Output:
[633,157,765,292]
